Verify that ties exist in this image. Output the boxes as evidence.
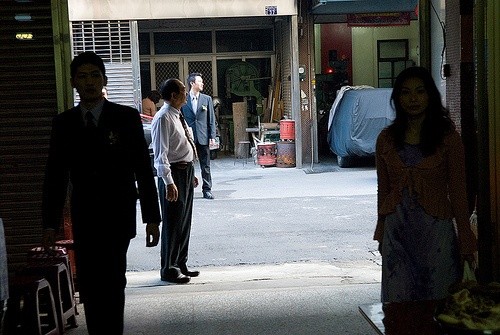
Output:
[180,114,197,159]
[193,97,197,115]
[85,112,97,148]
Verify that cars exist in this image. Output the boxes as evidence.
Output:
[335,155,349,169]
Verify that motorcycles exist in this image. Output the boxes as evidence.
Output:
[209,97,222,160]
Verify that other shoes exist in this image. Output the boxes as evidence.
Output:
[162,274,189,283]
[202,190,214,200]
[182,271,199,277]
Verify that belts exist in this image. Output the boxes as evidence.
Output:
[172,162,193,167]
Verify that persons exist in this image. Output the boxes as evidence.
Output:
[181,72,217,199]
[151,78,200,285]
[142,90,162,118]
[40,52,162,335]
[372,66,479,335]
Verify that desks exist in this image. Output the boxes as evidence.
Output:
[358,299,500,335]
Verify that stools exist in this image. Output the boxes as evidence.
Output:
[234,141,256,167]
[2,240,79,335]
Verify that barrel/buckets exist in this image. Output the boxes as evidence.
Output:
[257,142,276,165]
[276,141,296,168]
[280,120,295,139]
